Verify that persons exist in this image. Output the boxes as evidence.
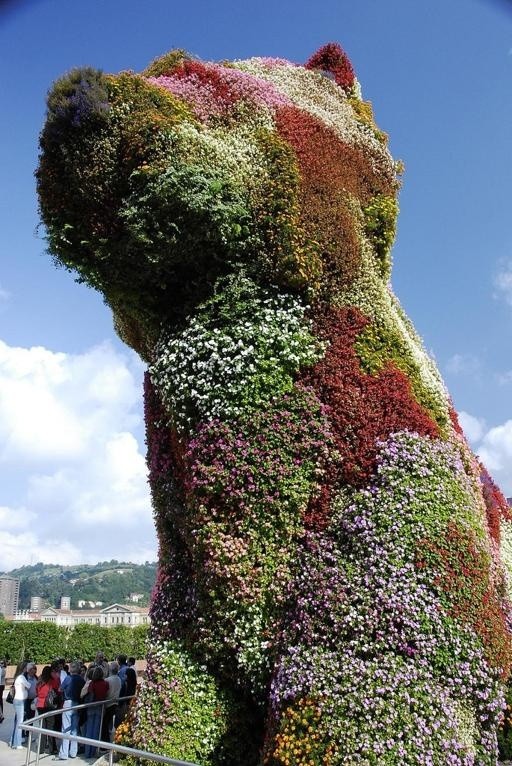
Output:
[7,651,139,761]
[0,663,7,723]
